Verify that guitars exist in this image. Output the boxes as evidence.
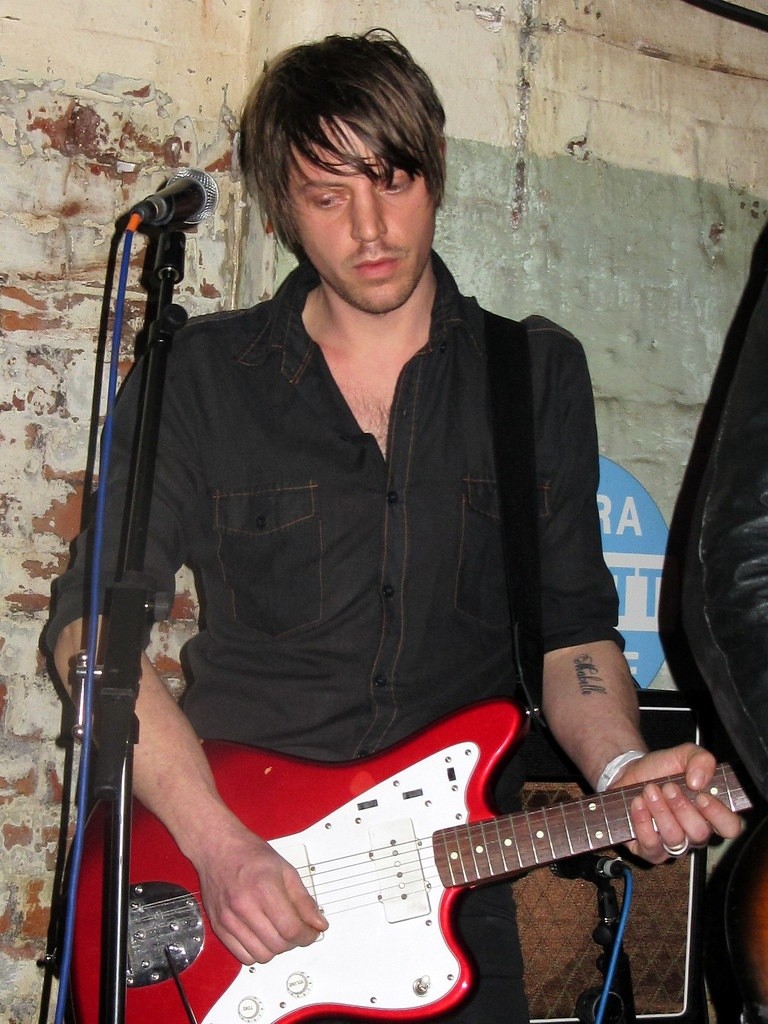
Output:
[62,692,755,1024]
[699,810,768,1024]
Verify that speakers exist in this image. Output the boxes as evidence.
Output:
[494,688,707,1024]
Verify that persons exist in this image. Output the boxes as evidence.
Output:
[38,26,742,1024]
[680,286,768,803]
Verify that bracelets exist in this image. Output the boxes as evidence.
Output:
[595,748,643,801]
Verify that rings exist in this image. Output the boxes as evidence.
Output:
[662,834,692,858]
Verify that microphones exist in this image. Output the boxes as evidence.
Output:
[131,168,219,225]
[549,853,628,878]
